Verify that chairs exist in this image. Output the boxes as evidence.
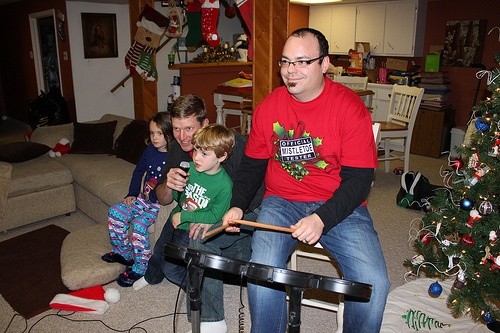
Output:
[289,243,344,333]
[333,74,369,91]
[212,86,252,134]
[371,84,424,188]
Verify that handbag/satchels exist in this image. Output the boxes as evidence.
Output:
[396,170,452,211]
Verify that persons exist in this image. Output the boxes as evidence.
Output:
[101,112,177,287]
[222,28,391,333]
[148,96,265,333]
[132,124,236,291]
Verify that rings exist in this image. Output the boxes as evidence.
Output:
[305,239,308,243]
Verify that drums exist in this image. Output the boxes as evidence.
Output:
[162,241,373,301]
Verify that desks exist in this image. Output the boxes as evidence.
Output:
[168,61,252,131]
[410,106,456,159]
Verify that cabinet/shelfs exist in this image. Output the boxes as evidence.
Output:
[355,0,426,57]
[308,0,358,55]
[366,85,405,153]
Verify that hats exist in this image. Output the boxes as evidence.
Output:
[49,285,120,315]
[48,137,71,158]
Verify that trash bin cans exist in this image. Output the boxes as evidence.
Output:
[449,128,467,158]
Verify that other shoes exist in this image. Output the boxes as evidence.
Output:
[101,253,142,287]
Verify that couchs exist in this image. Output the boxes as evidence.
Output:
[0,132,76,232]
[30,114,177,256]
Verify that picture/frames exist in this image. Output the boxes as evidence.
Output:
[80,12,118,59]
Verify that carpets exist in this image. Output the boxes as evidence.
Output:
[0,224,71,320]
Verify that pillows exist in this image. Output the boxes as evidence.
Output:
[67,120,118,155]
[116,120,148,165]
[0,141,52,164]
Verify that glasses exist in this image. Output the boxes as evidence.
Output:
[278,56,323,67]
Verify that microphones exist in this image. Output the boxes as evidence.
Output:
[179,161,191,201]
